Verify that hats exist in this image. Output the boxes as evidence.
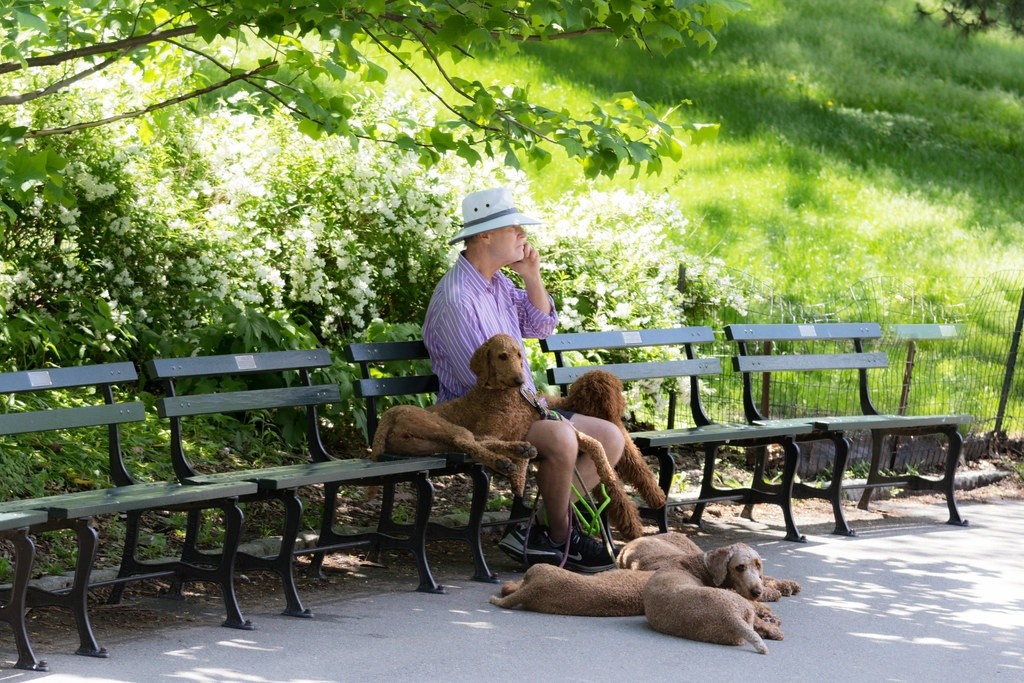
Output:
[448,187,543,245]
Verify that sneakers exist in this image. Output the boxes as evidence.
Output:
[498,523,564,566]
[544,528,619,573]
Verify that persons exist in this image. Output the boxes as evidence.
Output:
[421,186,625,571]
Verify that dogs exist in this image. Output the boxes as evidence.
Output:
[367,333,540,494]
[491,531,799,654]
[542,371,666,540]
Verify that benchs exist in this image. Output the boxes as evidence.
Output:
[348,338,621,582]
[0,361,257,658]
[538,322,815,542]
[142,346,446,618]
[724,322,974,538]
